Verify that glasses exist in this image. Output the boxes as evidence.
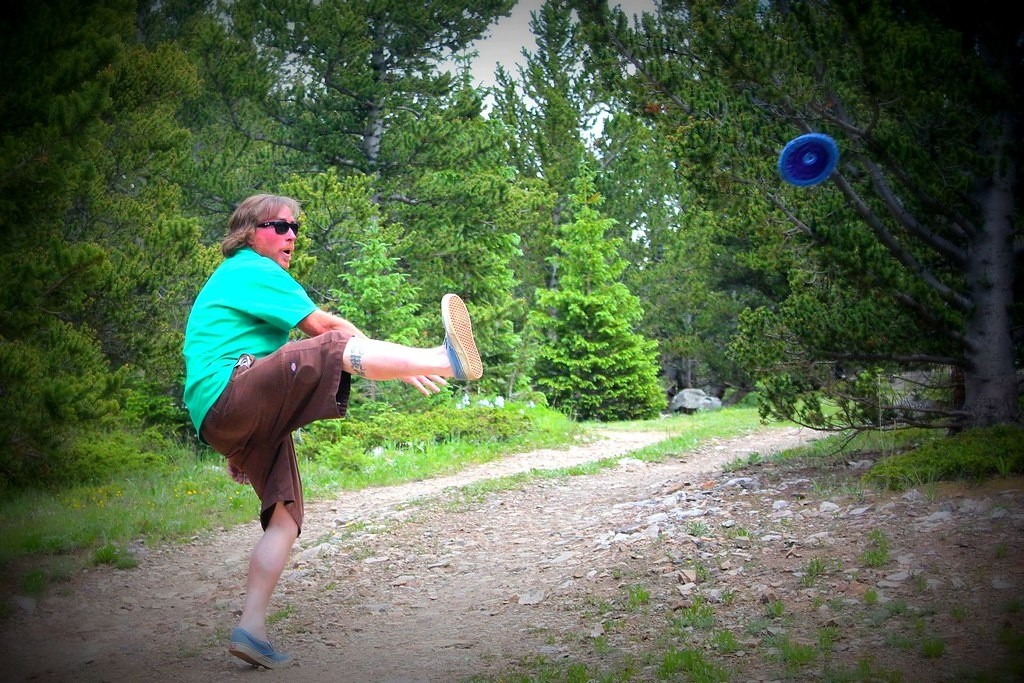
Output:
[256,220,299,238]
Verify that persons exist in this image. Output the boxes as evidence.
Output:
[183,193,483,670]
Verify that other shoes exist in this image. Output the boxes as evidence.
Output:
[441,294,484,380]
[228,627,293,669]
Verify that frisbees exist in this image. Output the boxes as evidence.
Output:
[777,134,838,189]
